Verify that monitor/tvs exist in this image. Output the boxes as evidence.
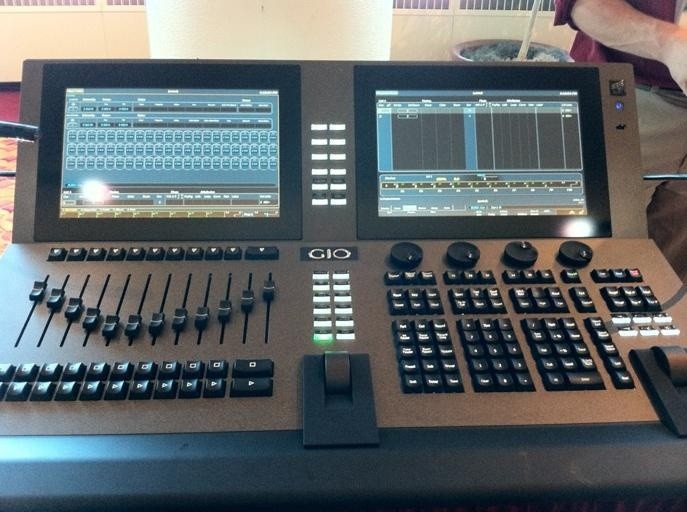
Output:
[354,65,612,241]
[33,61,304,243]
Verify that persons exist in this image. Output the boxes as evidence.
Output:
[551,0,686,280]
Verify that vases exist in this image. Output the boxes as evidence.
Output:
[452,39,575,62]
